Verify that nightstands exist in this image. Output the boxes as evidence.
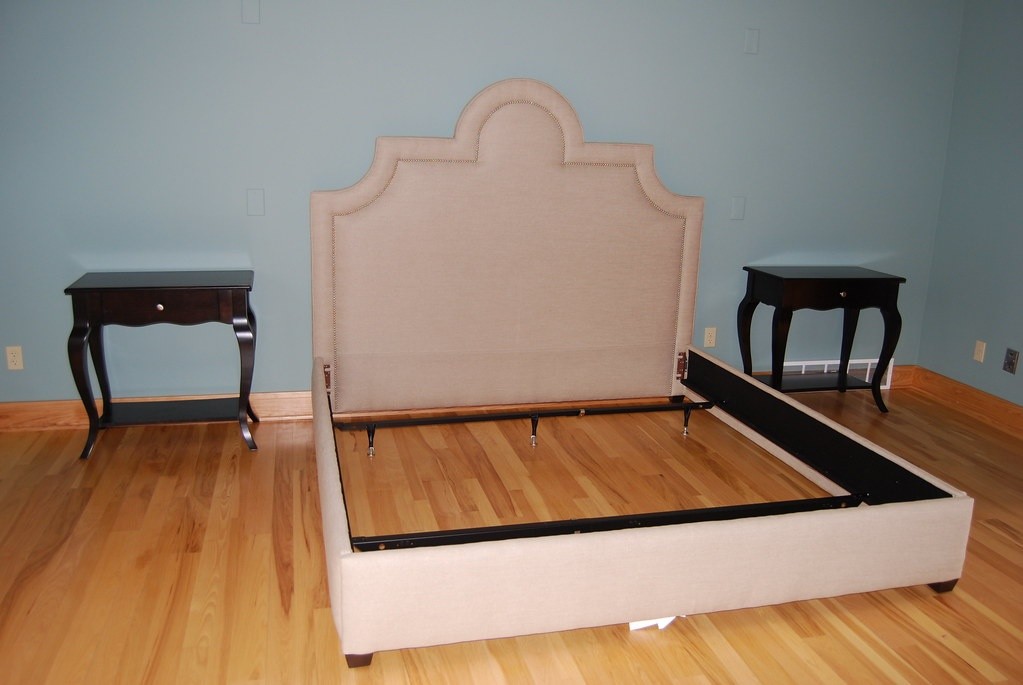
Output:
[65,269,261,460]
[735,266,907,413]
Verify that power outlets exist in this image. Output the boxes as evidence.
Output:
[704,327,716,348]
[1003,348,1019,375]
[973,340,987,364]
[6,346,24,370]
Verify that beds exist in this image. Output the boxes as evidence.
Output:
[308,77,976,670]
